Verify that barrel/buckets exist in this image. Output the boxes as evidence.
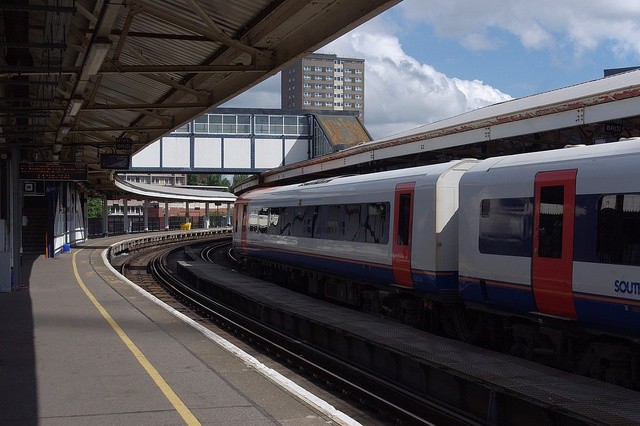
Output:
[63,243,70,252]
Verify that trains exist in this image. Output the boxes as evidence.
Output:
[230,66,638,351]
